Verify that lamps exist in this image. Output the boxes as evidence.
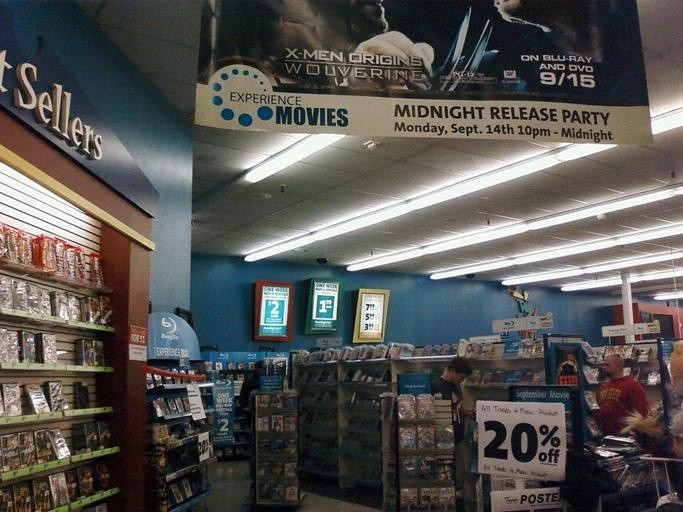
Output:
[193,104,683,302]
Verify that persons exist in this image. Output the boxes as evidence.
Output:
[431,354,476,510]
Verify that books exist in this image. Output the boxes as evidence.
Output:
[407,329,546,387]
[291,341,414,498]
[384,395,455,512]
[0,220,121,512]
[145,353,298,512]
[547,332,682,496]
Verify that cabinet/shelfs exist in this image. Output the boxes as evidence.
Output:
[142,313,682,510]
[3,142,134,512]
